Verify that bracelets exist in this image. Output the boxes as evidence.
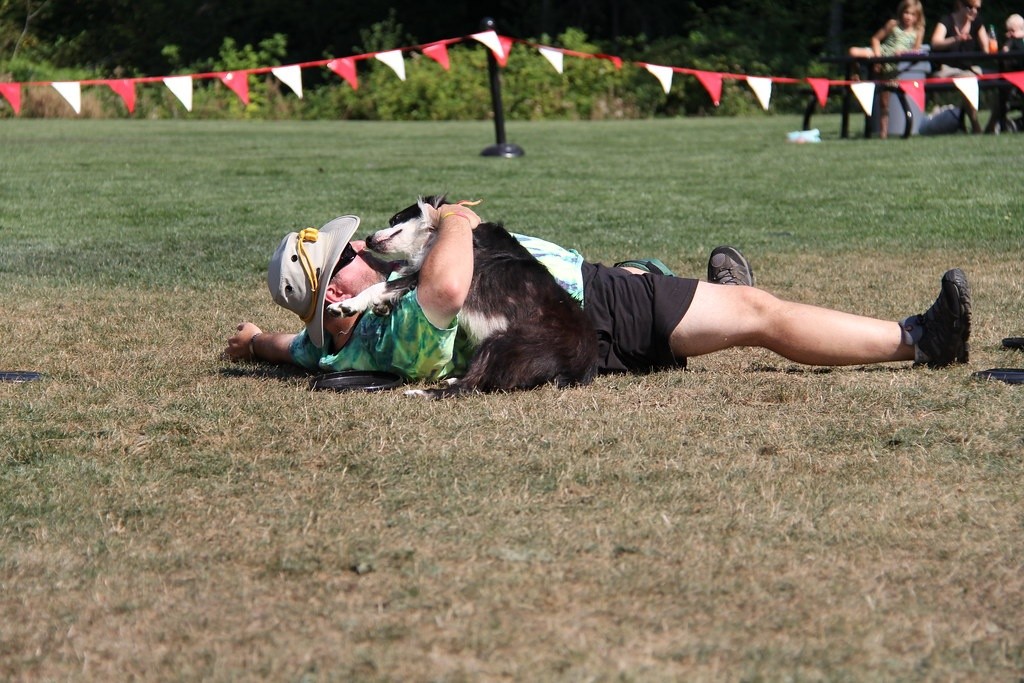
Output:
[249,332,262,355]
[442,212,470,221]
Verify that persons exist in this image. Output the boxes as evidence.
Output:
[930,0,989,134]
[1001,14,1024,52]
[228,204,972,383]
[849,0,925,139]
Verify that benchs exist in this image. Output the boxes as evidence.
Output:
[802,80,1013,137]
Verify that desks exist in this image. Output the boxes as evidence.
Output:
[802,51,1024,139]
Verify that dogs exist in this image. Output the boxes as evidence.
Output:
[325,192,604,400]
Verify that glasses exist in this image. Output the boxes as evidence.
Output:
[328,242,357,284]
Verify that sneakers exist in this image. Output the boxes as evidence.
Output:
[707,245,755,288]
[899,266,973,368]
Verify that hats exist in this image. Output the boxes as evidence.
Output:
[268,214,361,349]
